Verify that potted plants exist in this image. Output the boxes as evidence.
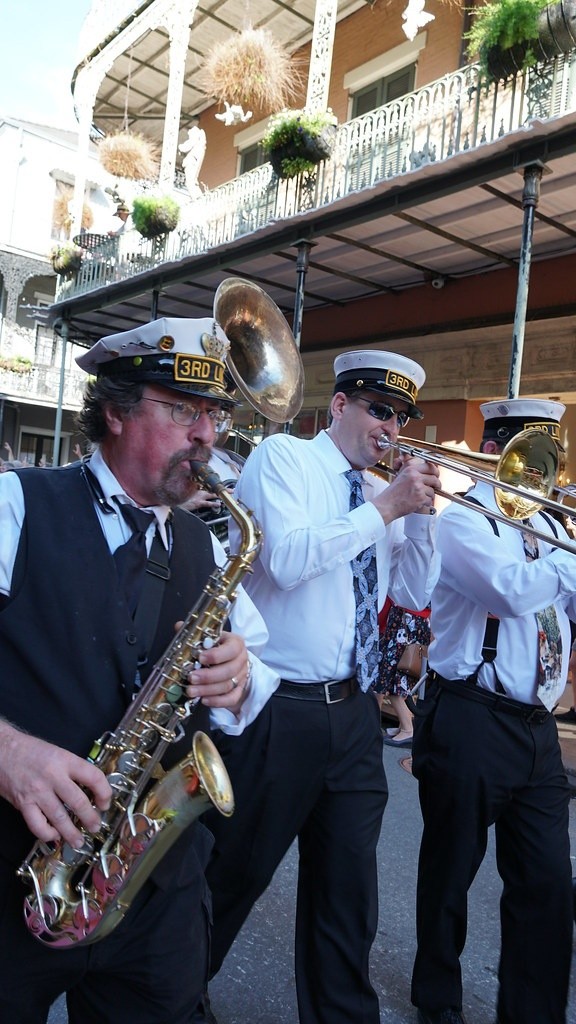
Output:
[51,246,81,272]
[132,200,176,238]
[460,1,576,98]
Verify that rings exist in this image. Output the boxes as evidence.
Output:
[232,678,238,687]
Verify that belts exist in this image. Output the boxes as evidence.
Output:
[272,678,360,704]
[438,676,553,724]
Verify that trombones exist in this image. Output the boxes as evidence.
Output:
[374,428,576,555]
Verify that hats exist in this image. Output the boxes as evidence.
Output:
[333,349,427,420]
[479,398,567,452]
[75,317,244,407]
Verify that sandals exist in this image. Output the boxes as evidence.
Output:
[400,757,413,773]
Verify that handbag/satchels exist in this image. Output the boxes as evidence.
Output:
[397,644,427,678]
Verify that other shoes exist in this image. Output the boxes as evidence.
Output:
[417,1007,467,1024]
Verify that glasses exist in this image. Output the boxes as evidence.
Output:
[137,396,233,432]
[351,395,410,429]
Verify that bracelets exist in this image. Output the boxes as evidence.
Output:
[245,659,253,692]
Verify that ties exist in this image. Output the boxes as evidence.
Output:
[520,518,563,713]
[110,495,156,620]
[343,470,379,694]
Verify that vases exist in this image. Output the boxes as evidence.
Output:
[267,130,330,178]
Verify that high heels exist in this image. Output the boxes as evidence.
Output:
[384,738,413,748]
[384,734,396,739]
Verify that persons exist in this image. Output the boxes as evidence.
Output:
[2,317,281,1024]
[209,348,440,1024]
[179,126,206,201]
[378,595,576,748]
[108,205,137,237]
[411,400,576,1023]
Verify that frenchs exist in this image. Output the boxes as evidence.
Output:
[206,275,306,449]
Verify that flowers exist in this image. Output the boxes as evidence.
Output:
[257,117,334,178]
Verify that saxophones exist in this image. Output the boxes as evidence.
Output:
[15,459,268,950]
